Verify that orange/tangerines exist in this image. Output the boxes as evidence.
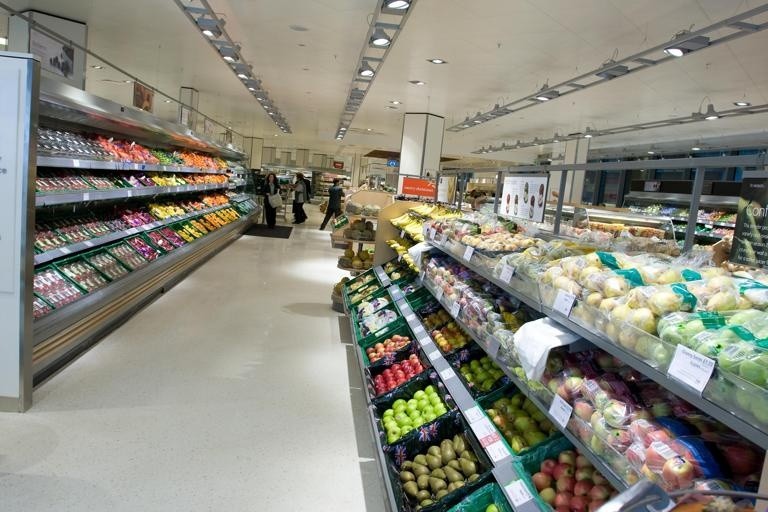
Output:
[152,150,171,165]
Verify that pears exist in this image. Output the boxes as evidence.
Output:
[399,433,482,511]
[486,394,557,457]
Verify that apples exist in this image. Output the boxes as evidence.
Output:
[486,504,499,512]
[366,254,523,445]
[540,344,756,512]
[537,250,767,425]
[459,231,535,252]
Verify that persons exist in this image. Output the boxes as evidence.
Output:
[319,179,345,230]
[262,174,281,228]
[289,172,312,224]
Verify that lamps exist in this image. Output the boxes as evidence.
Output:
[458,103,517,130]
[593,56,631,82]
[535,92,552,112]
[701,99,721,125]
[196,16,292,143]
[332,1,417,142]
[660,42,689,65]
[475,131,597,150]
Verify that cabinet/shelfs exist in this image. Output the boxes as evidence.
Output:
[1,51,262,413]
[331,213,378,272]
[339,201,768,512]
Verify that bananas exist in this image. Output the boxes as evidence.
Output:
[385,201,464,274]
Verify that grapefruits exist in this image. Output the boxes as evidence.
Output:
[336,201,380,296]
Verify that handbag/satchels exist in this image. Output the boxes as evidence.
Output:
[267,189,283,208]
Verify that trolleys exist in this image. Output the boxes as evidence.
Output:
[276,189,292,223]
